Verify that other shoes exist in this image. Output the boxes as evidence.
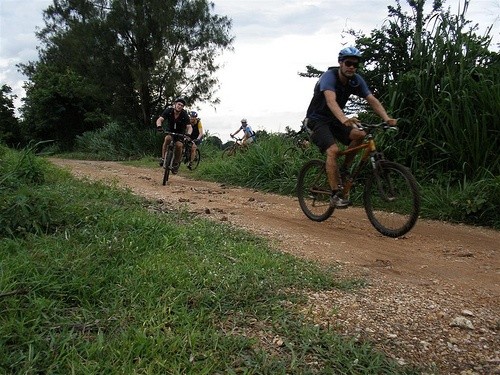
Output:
[187,162,192,168]
[330,192,349,206]
[173,166,178,173]
[159,159,163,164]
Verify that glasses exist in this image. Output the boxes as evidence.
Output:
[345,60,359,68]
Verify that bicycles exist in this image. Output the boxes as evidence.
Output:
[295,118,421,238]
[222,133,254,159]
[155,128,201,186]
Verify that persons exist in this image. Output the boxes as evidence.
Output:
[156,97,193,174]
[303,47,397,206]
[231,118,256,150]
[188,111,203,166]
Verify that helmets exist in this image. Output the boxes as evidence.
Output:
[175,97,187,106]
[339,47,362,60]
[190,112,197,117]
[241,119,246,123]
